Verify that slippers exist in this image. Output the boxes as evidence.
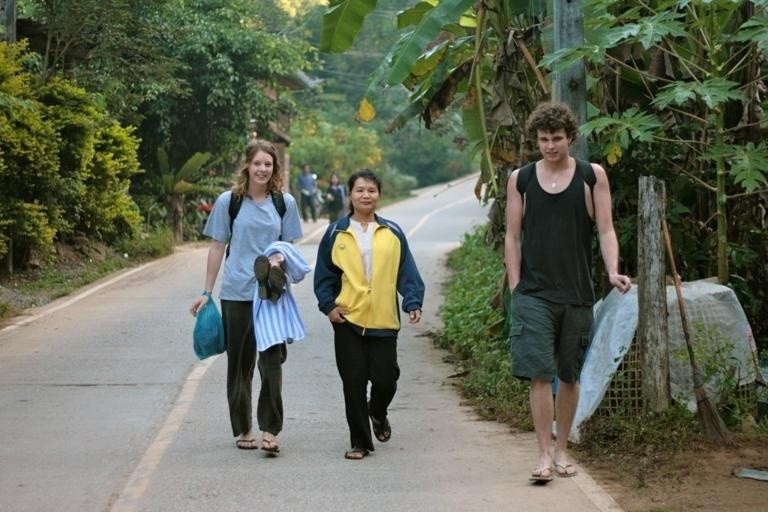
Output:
[261,438,280,453]
[530,467,553,480]
[236,439,257,449]
[368,400,392,442]
[345,446,369,458]
[554,462,577,477]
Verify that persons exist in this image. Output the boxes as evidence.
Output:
[297,164,347,224]
[505,102,630,482]
[189,140,303,452]
[314,170,426,460]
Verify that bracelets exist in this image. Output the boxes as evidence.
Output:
[203,290,212,296]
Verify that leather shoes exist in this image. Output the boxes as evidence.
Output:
[269,265,286,302]
[254,255,270,299]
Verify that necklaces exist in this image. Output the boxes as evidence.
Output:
[548,173,565,188]
